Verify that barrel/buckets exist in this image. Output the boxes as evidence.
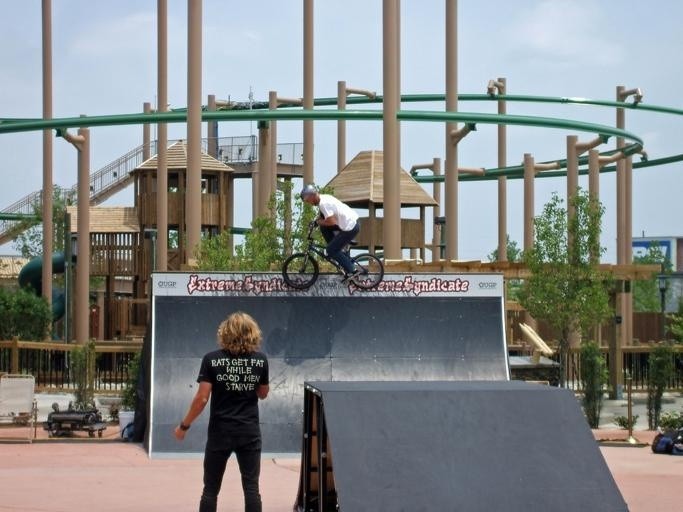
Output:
[118,410,135,439]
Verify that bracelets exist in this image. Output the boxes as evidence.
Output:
[180,421,190,431]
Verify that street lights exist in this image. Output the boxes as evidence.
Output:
[654,263,670,347]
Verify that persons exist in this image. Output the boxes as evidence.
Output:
[174,310,270,512]
[300,183,361,283]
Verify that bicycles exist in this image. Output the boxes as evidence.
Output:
[281,223,384,291]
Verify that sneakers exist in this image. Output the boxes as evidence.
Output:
[341,270,360,282]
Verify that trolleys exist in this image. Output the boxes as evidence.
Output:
[45,422,106,440]
[41,399,101,423]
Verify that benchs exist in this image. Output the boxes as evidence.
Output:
[44,410,107,437]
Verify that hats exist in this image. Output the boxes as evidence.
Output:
[300,184,315,195]
